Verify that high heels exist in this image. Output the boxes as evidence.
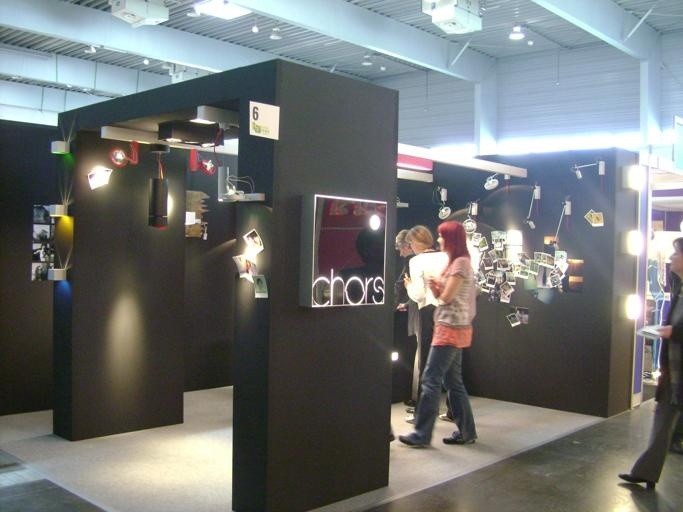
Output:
[619,474,655,491]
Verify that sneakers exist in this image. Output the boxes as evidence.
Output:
[399,400,475,447]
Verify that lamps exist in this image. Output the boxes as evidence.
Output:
[215,164,265,202]
[270,19,281,40]
[432,159,607,252]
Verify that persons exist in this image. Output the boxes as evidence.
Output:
[246,231,262,249]
[34,206,51,281]
[669,405,683,453]
[474,239,563,326]
[618,237,683,490]
[255,279,267,293]
[395,220,478,448]
[245,260,252,277]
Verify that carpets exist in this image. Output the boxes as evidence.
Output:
[0,384,605,512]
